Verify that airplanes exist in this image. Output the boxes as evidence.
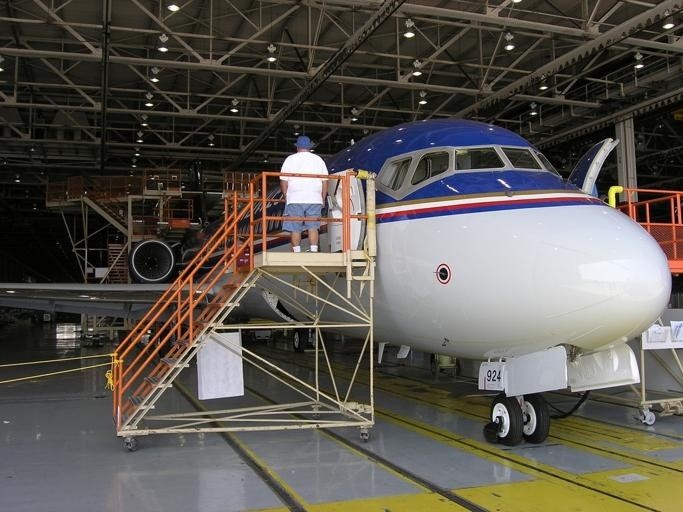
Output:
[1,117,672,448]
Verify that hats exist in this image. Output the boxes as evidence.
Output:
[294,136,313,148]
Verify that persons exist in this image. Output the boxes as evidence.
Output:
[280,136,329,253]
